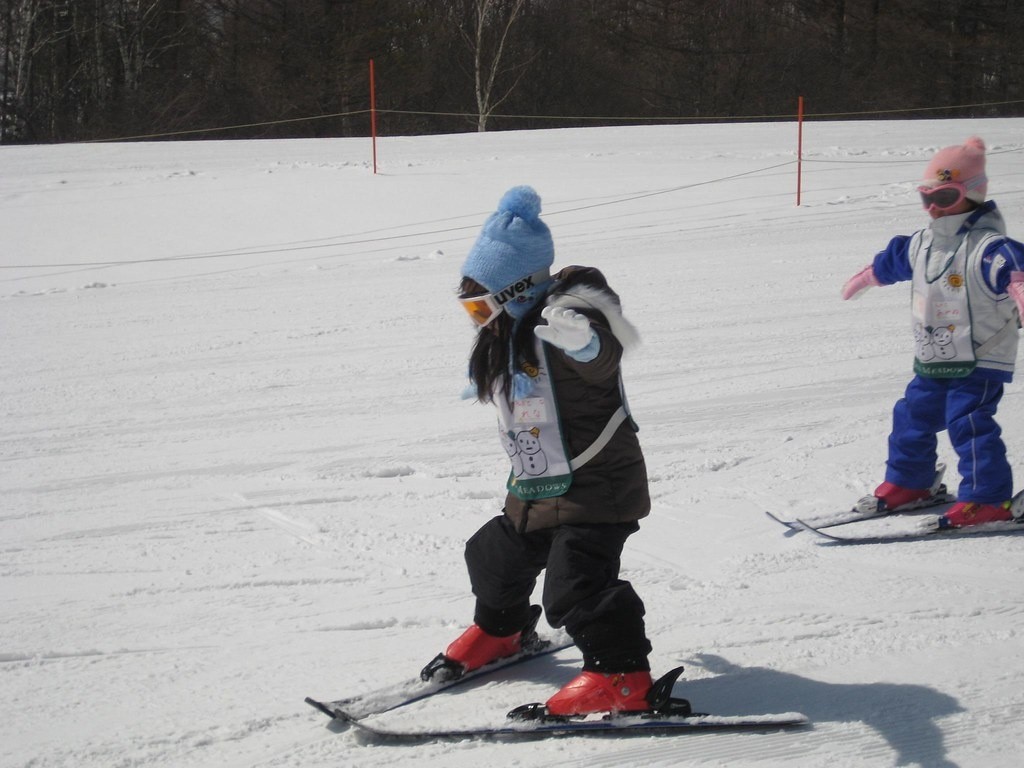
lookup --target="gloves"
[843,265,883,300]
[533,305,591,353]
[1006,272,1024,328]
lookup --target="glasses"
[458,293,504,328]
[916,183,966,211]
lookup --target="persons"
[444,186,655,718]
[838,136,1023,528]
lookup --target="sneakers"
[448,597,523,673]
[875,481,931,512]
[543,661,657,717]
[939,495,1012,528]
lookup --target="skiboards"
[304,623,811,734]
[765,492,1023,540]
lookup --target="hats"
[918,136,989,204]
[460,184,554,320]
